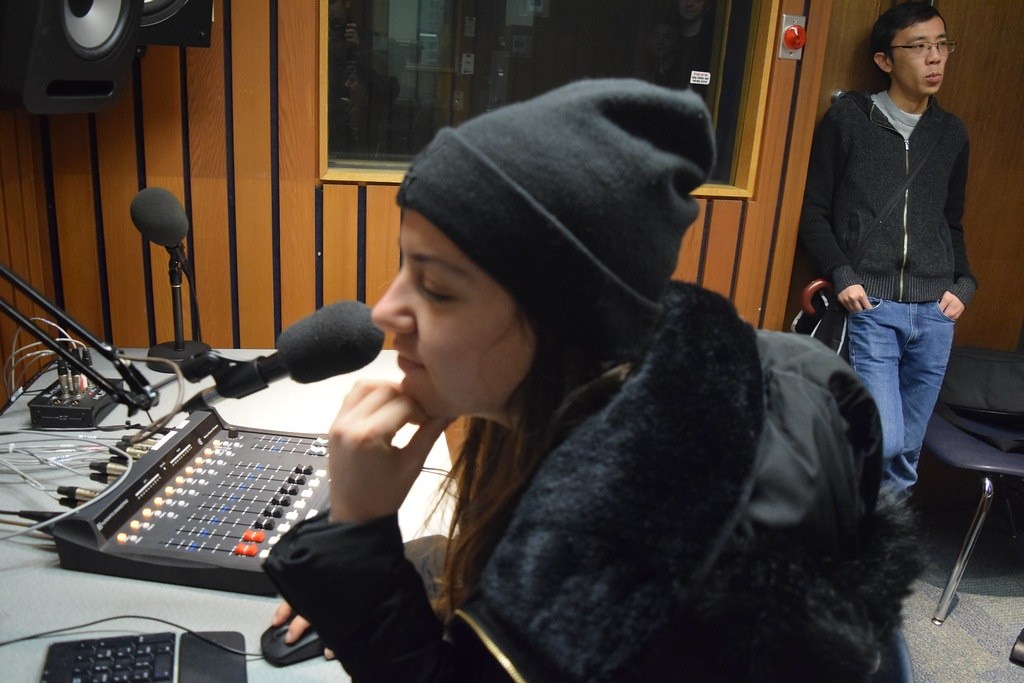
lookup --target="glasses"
[885,40,957,54]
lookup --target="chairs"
[924,410,1024,628]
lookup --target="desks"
[1,342,458,682]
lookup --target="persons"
[799,2,980,506]
[623,18,682,82]
[328,0,406,154]
[676,0,714,103]
[263,76,923,682]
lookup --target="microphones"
[131,188,193,282]
[180,300,385,414]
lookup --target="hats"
[397,77,715,350]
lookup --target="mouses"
[260,608,327,667]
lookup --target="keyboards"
[39,631,248,683]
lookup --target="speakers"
[142,0,214,48]
[0,0,145,114]
